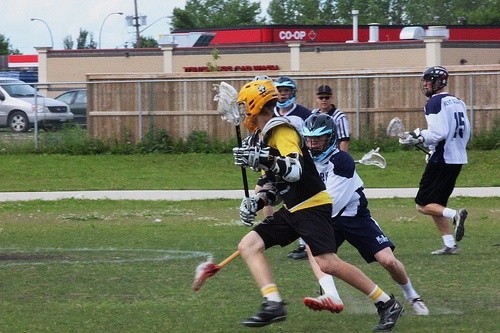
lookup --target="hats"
[315,84,333,98]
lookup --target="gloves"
[398,126,425,146]
[232,142,272,173]
[239,194,260,227]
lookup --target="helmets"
[300,112,338,154]
[419,65,449,98]
[235,74,280,133]
[274,75,298,109]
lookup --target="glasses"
[318,96,330,100]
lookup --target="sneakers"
[430,244,459,255]
[452,208,468,242]
[241,296,290,327]
[286,244,309,260]
[404,292,430,316]
[370,293,406,332]
[303,294,344,313]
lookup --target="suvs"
[0,68,39,88]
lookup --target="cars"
[0,77,75,134]
[41,89,89,134]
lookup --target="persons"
[398,66,470,256]
[233,74,431,332]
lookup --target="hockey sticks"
[190,250,240,291]
[384,117,431,156]
[213,81,251,219]
[352,147,390,170]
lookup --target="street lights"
[30,18,54,52]
[133,0,175,47]
[98,12,124,49]
